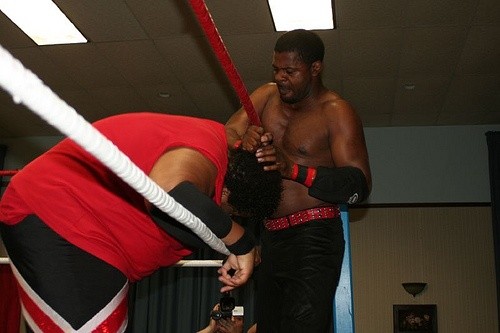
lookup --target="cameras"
[211,295,244,321]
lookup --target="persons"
[194,302,245,333]
[246,322,257,333]
[0,111,284,333]
[224,29,374,333]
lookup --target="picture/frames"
[393,304,438,333]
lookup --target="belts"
[262,205,340,232]
